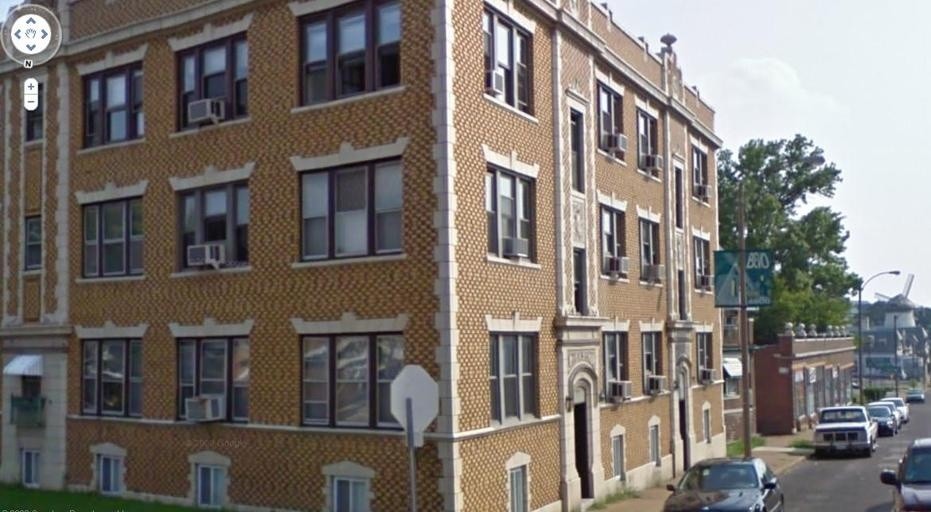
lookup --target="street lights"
[857,272,897,401]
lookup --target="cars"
[813,396,908,455]
[906,390,925,402]
[880,438,931,511]
[664,459,784,512]
